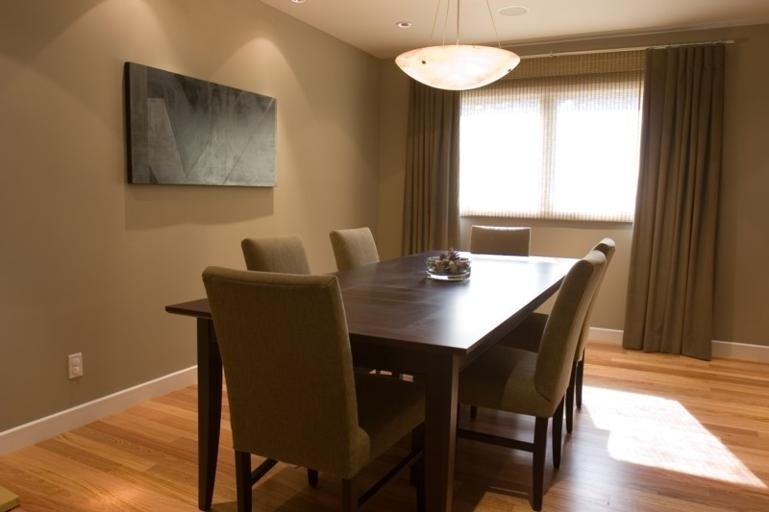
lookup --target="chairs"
[329,227,390,267]
[470,226,533,254]
[202,258,426,512]
[469,237,616,433]
[237,238,328,274]
[460,249,606,510]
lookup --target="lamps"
[394,0,523,95]
